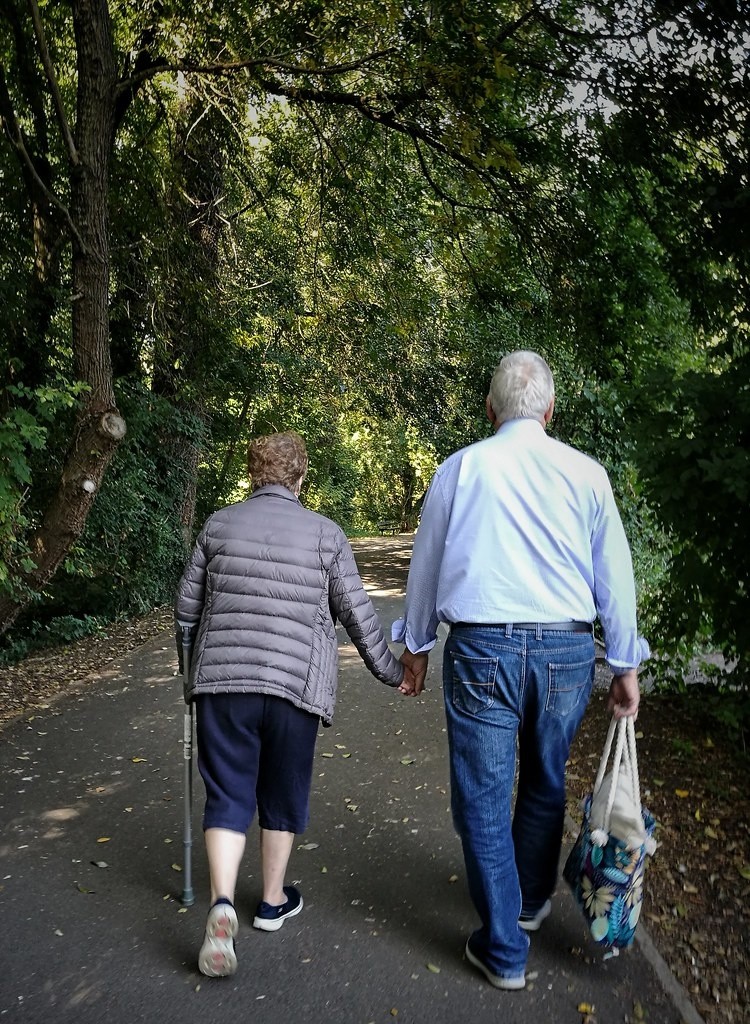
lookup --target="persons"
[390,350,652,989]
[175,429,427,978]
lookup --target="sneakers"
[465,934,526,990]
[518,899,552,930]
[252,886,304,932]
[198,898,238,977]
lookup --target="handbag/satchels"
[561,707,657,962]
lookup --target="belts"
[451,621,592,632]
